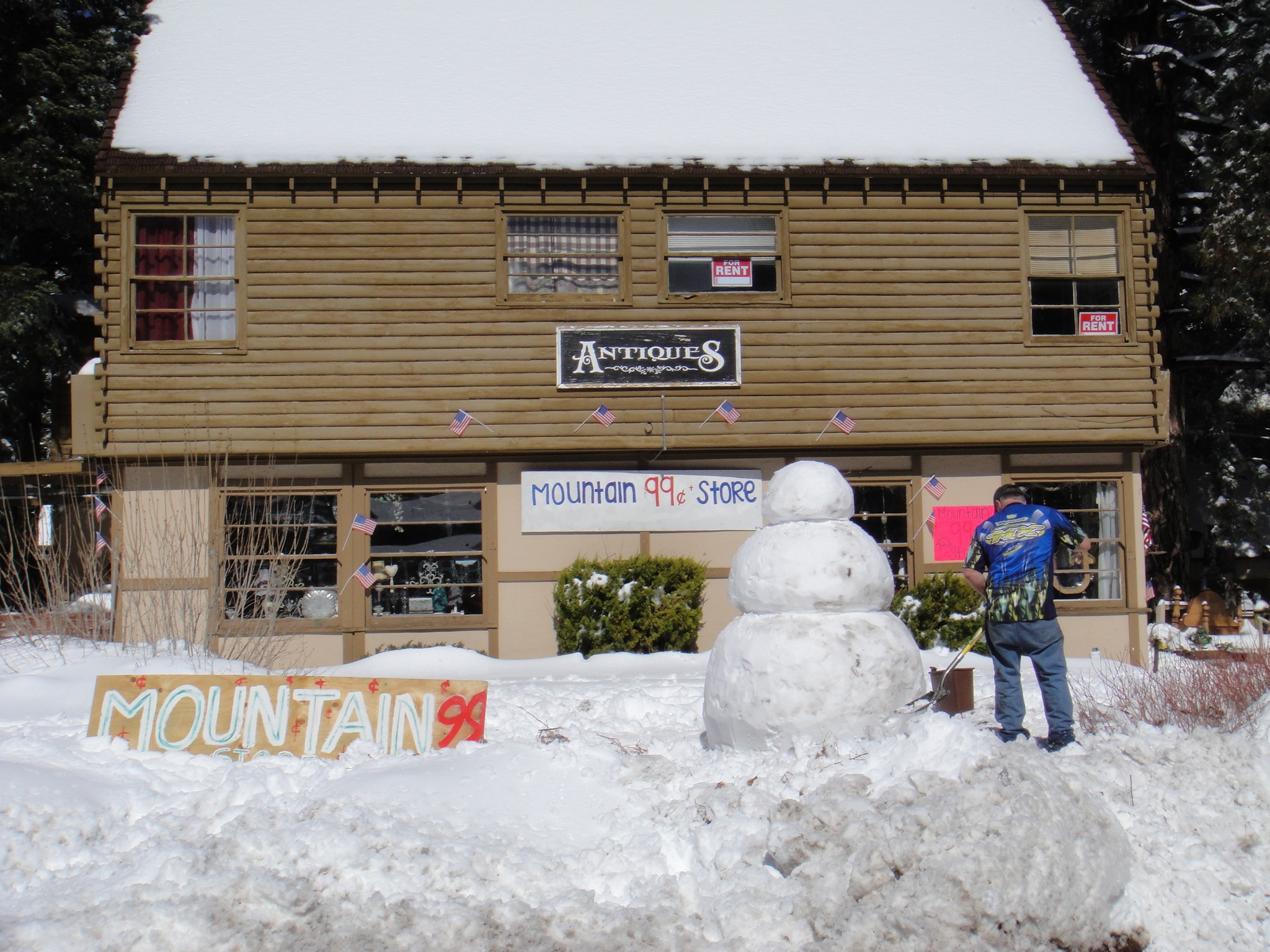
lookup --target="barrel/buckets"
[928,666,974,717]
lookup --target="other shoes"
[1047,730,1075,752]
[999,727,1029,741]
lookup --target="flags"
[93,467,109,489]
[927,511,937,531]
[92,498,106,521]
[92,535,107,558]
[354,563,379,589]
[593,403,617,428]
[924,475,948,500]
[352,513,378,537]
[716,400,741,426]
[1143,500,1154,555]
[447,411,473,437]
[1146,579,1156,602]
[831,410,857,435]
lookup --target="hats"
[993,483,1023,514]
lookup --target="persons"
[963,483,1091,755]
